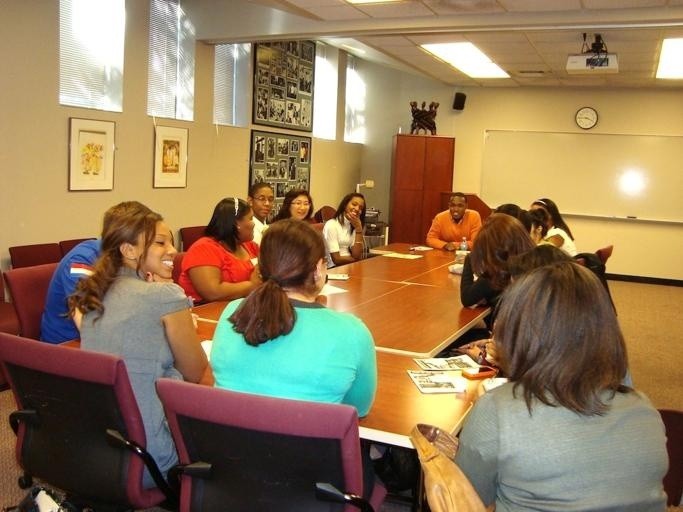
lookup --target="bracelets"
[355,231,363,234]
[355,242,363,244]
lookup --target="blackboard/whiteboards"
[479,129,683,224]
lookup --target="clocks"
[575,106,598,129]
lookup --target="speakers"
[453,93,466,110]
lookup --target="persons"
[209,219,378,502]
[454,261,669,511]
[271,187,313,219]
[492,203,531,237]
[321,193,365,267]
[531,198,578,257]
[41,201,149,345]
[506,245,572,283]
[460,212,536,309]
[58,207,208,491]
[412,353,478,369]
[248,182,275,275]
[528,208,557,246]
[180,198,261,307]
[426,191,482,251]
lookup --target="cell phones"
[463,365,500,379]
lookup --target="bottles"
[460,237,468,251]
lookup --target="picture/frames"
[153,125,190,189]
[249,128,313,225]
[66,117,116,191]
[254,40,316,134]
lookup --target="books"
[320,285,348,294]
[407,370,464,394]
[382,252,424,260]
[412,246,433,251]
[328,273,349,281]
[456,250,467,256]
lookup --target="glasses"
[285,201,312,207]
[252,196,274,202]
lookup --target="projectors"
[566,53,618,74]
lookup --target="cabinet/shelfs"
[388,132,456,245]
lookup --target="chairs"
[1,330,176,510]
[7,243,62,268]
[60,238,97,261]
[179,225,209,251]
[3,262,59,341]
[153,375,388,511]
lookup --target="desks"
[56,240,507,511]
